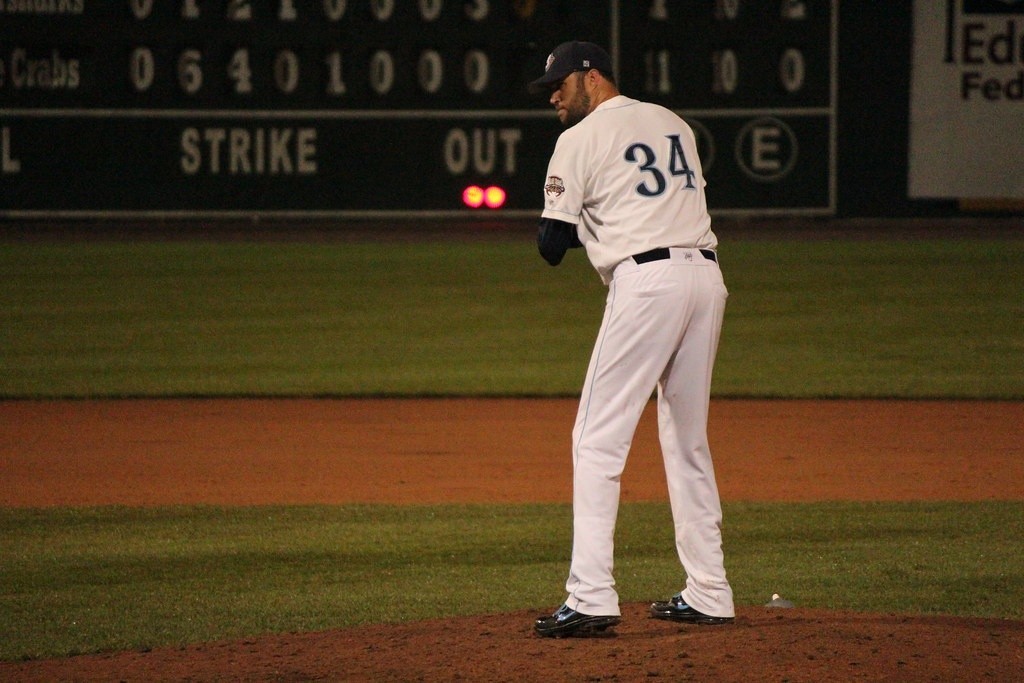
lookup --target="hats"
[531,40,616,104]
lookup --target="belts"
[631,248,718,265]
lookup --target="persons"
[527,40,737,634]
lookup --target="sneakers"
[648,593,735,624]
[532,601,622,636]
